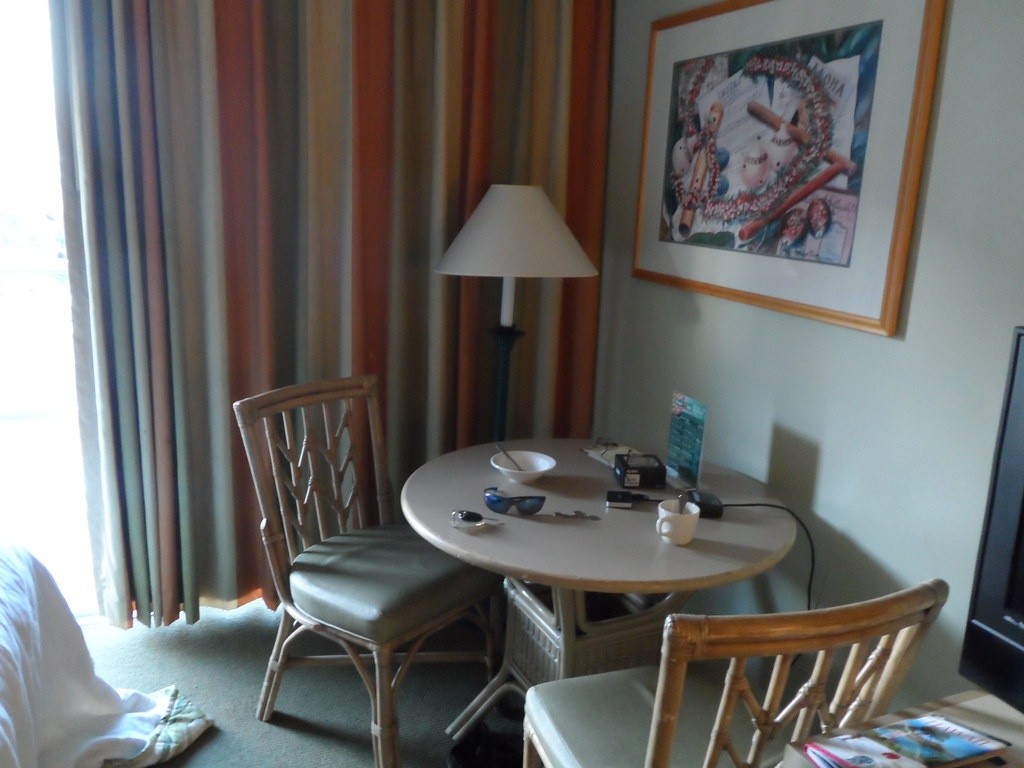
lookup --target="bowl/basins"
[490,451,557,483]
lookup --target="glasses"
[483,487,546,516]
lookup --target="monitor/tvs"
[960,328,1024,714]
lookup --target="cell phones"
[605,490,633,508]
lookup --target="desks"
[400,438,797,742]
[785,686,1024,768]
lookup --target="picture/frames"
[631,0,943,336]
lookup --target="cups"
[655,500,700,546]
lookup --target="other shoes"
[447,721,523,767]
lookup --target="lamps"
[435,181,600,443]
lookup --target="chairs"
[523,578,949,768]
[232,374,503,768]
